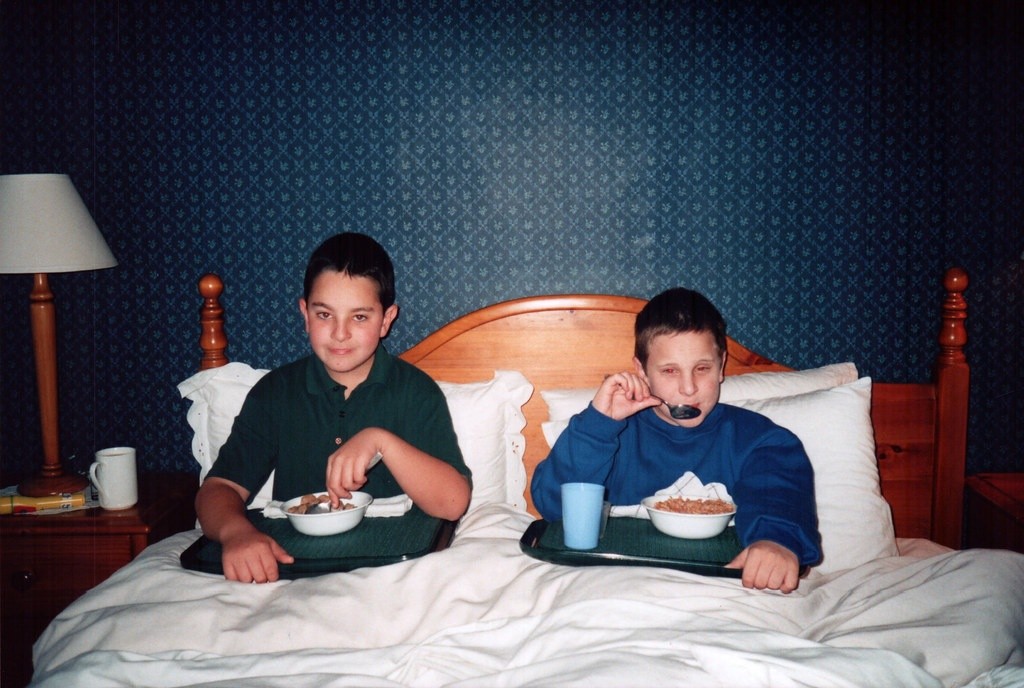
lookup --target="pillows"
[541,362,857,422]
[542,376,900,576]
[177,361,534,515]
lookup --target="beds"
[30,230,1024,688]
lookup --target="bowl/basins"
[280,491,373,537]
[641,494,737,539]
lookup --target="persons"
[195,232,473,585]
[529,286,819,594]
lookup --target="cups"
[89,447,139,511]
[561,482,605,551]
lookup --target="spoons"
[305,452,382,514]
[604,373,701,419]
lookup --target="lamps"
[0,172,117,498]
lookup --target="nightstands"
[964,472,1024,555]
[0,472,199,688]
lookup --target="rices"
[653,497,734,515]
